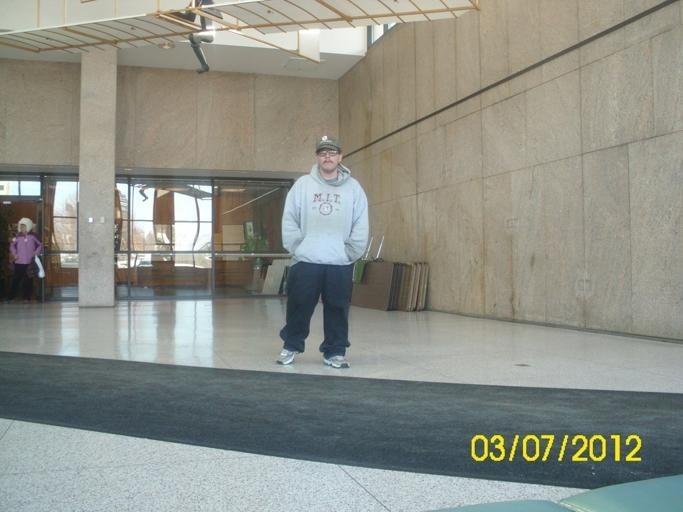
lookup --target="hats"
[316,135,341,152]
[17,217,33,233]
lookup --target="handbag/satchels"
[32,254,45,279]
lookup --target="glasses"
[317,150,338,156]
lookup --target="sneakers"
[323,355,350,369]
[276,349,300,366]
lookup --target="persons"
[9,217,41,302]
[275,136,368,367]
